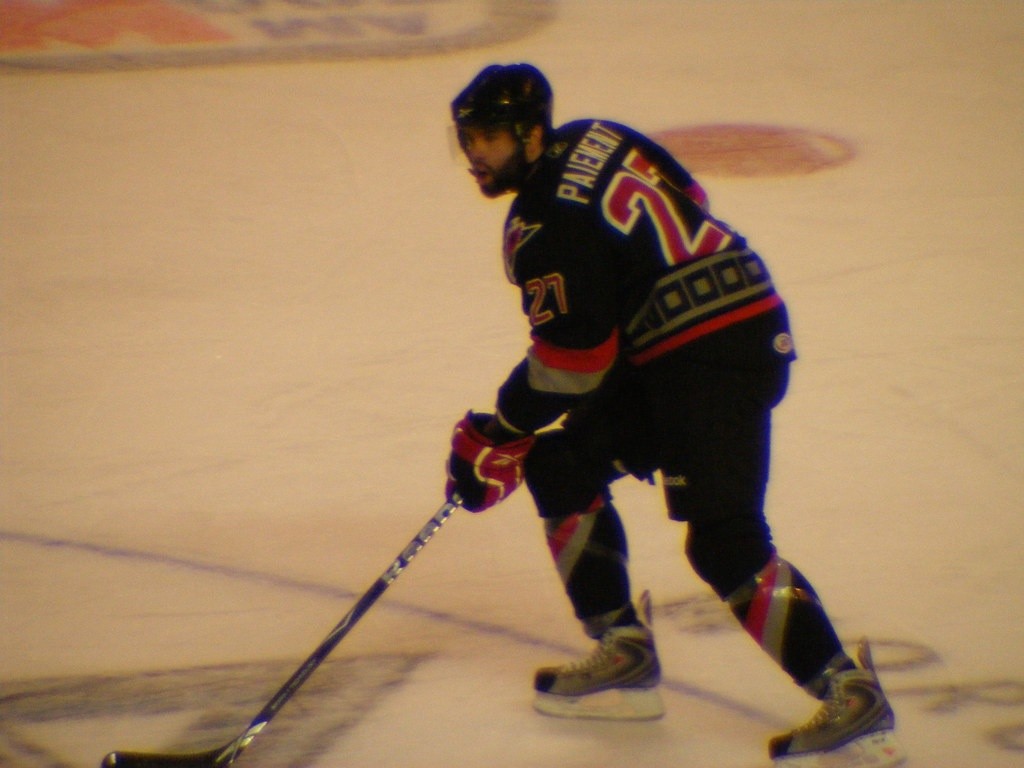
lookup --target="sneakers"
[526,590,666,721]
[768,636,904,768]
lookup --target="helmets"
[450,63,552,144]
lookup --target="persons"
[445,64,895,759]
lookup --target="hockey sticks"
[99,489,465,768]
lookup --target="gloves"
[445,410,536,514]
